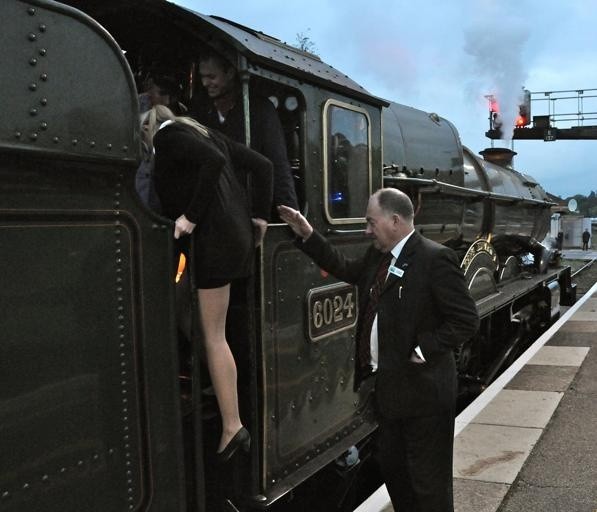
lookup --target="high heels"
[211,427,250,468]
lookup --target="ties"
[357,250,394,369]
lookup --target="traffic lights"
[533,116,549,128]
[492,97,502,129]
[516,90,530,125]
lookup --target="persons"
[138,105,273,464]
[190,49,299,216]
[583,228,590,251]
[277,188,482,512]
[135,64,188,218]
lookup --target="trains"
[0,0,582,511]
